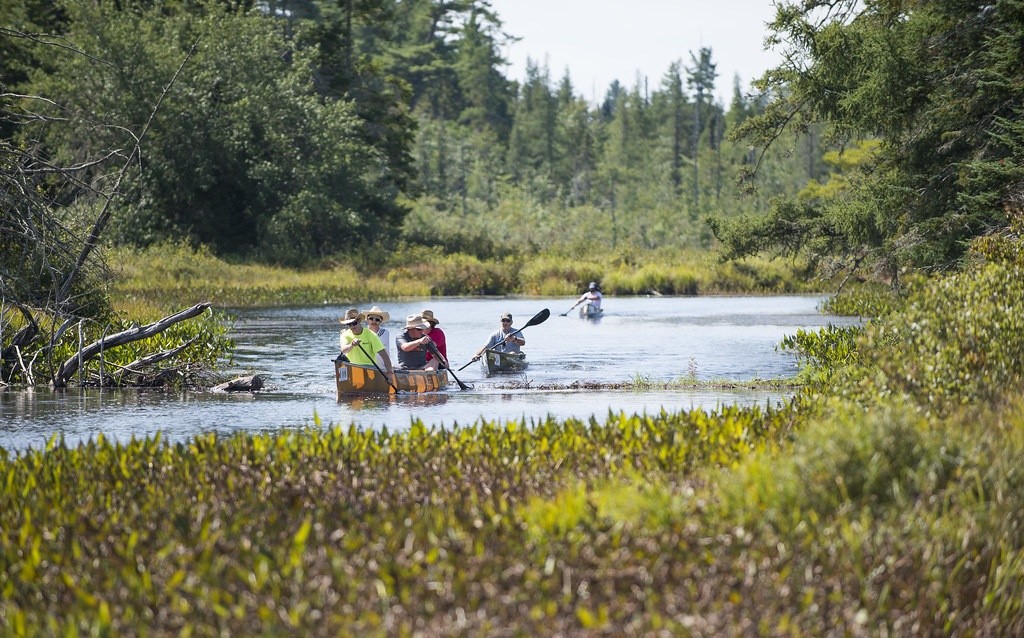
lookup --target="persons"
[577,282,602,312]
[472,312,526,362]
[338,305,449,386]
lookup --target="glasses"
[415,328,422,330]
[502,319,510,322]
[347,321,357,327]
[368,317,381,322]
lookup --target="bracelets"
[387,371,394,374]
[512,337,517,342]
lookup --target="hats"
[339,308,362,325]
[361,306,390,323]
[401,315,430,330]
[420,310,439,324]
[589,282,597,289]
[500,313,512,321]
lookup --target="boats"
[479,349,530,378]
[579,304,603,319]
[330,359,448,396]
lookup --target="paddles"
[356,342,402,396]
[455,308,551,372]
[557,296,588,318]
[426,340,473,392]
